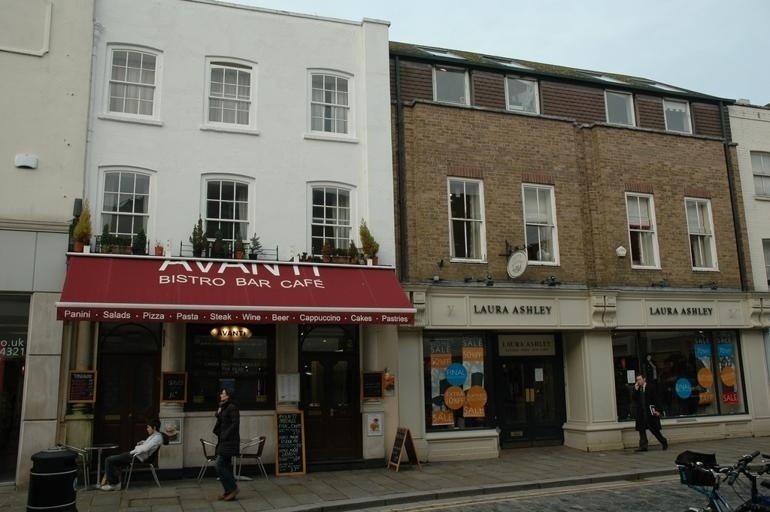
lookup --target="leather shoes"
[634,439,667,451]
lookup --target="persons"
[632,374,669,451]
[95,418,164,491]
[212,386,240,500]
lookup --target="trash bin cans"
[26,447,82,512]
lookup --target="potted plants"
[321,216,379,266]
[188,212,264,259]
[72,198,164,256]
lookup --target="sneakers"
[101,483,121,491]
[218,488,240,501]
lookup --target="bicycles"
[675,445,770,512]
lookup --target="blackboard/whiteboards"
[388,428,408,466]
[160,371,187,404]
[68,370,97,404]
[361,369,386,400]
[274,409,307,475]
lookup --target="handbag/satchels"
[675,451,717,487]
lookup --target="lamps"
[499,239,528,280]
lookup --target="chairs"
[123,444,161,492]
[55,442,89,491]
[236,435,269,482]
[197,438,217,485]
